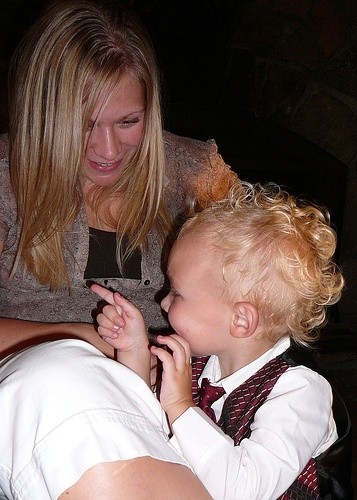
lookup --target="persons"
[90,181,343,500]
[0,0,248,500]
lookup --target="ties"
[198,377,226,423]
